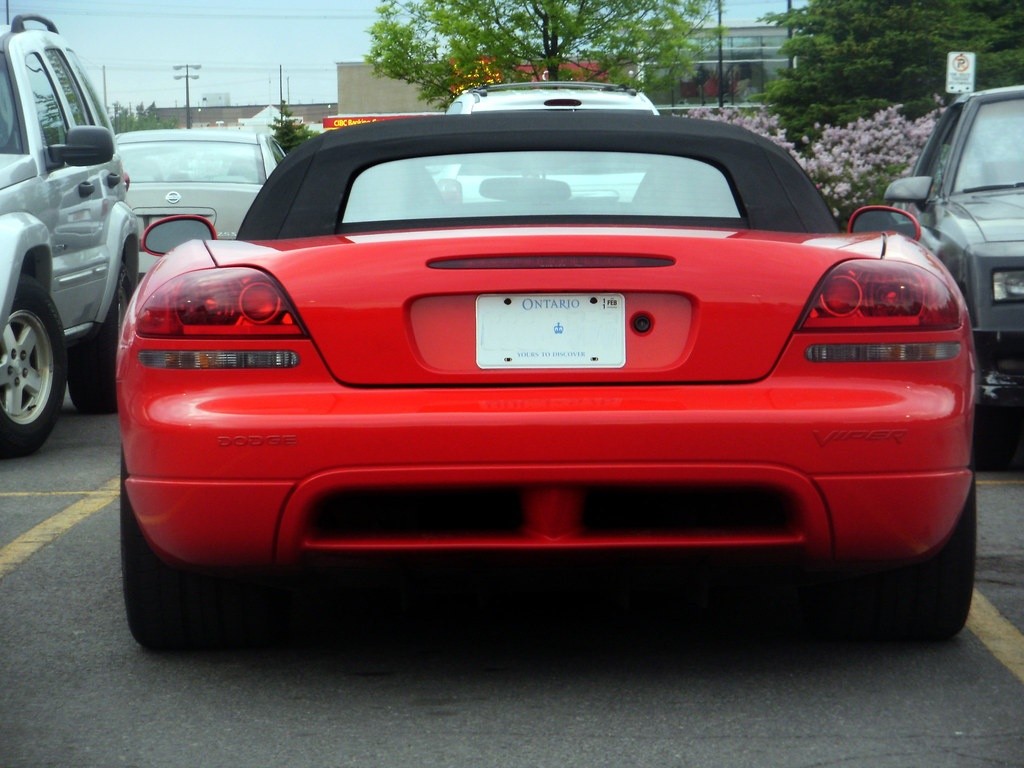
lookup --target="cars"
[107,122,286,283]
[889,84,1024,476]
[436,80,660,206]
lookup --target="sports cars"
[114,113,980,661]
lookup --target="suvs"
[1,10,142,460]
